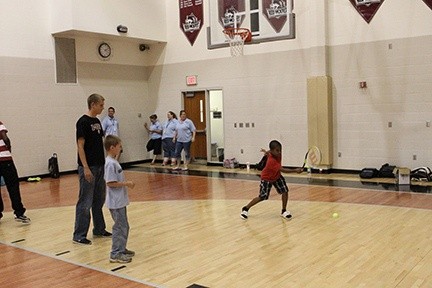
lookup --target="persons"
[172,110,197,171]
[1,114,31,223]
[161,112,179,166]
[104,135,136,262]
[144,115,163,165]
[73,94,113,245]
[242,140,292,219]
[101,107,123,161]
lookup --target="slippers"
[280,209,292,220]
[240,207,249,219]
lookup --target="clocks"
[96,41,114,61]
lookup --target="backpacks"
[256,150,268,171]
[48,153,60,178]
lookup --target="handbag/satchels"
[379,163,395,189]
[224,158,238,169]
[395,167,410,184]
[360,167,378,185]
[411,167,432,192]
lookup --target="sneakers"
[121,250,134,257]
[110,254,132,263]
[93,231,113,236]
[14,215,30,224]
[72,237,91,245]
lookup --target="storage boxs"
[392,167,411,184]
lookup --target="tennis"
[332,213,339,219]
